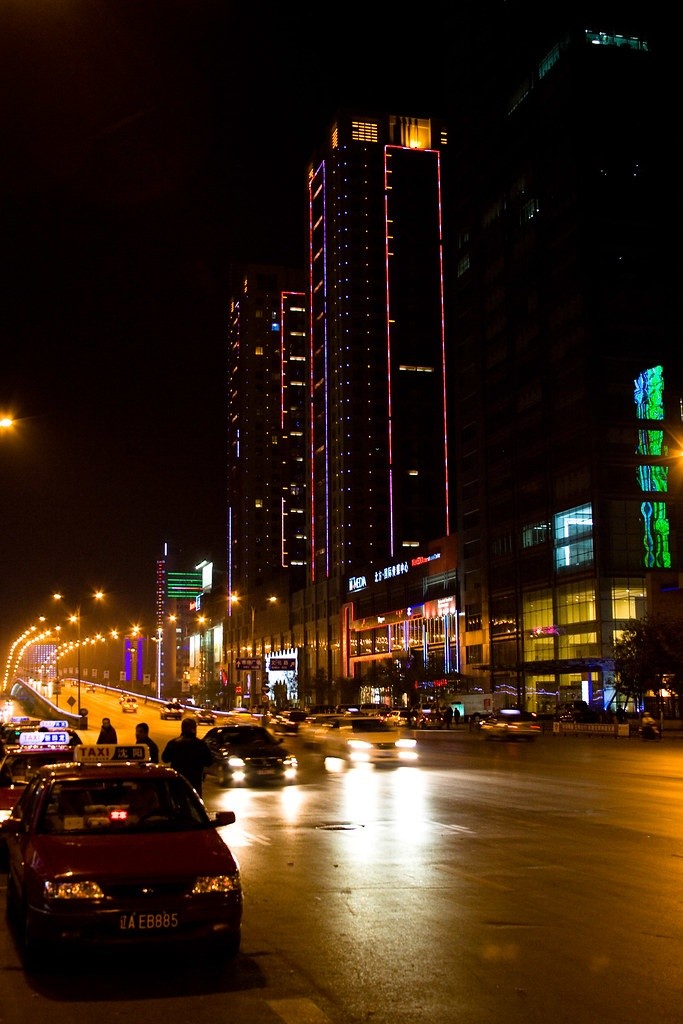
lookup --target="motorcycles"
[639,726,662,741]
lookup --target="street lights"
[53,592,104,713]
[2,617,61,707]
[131,624,156,696]
[54,628,119,686]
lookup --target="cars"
[480,710,543,741]
[0,744,243,977]
[201,724,298,786]
[336,702,444,730]
[0,718,84,822]
[196,711,217,725]
[159,703,183,720]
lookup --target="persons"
[436,709,443,721]
[444,707,453,728]
[96,717,117,747]
[605,708,614,725]
[161,718,214,808]
[642,711,656,729]
[133,723,158,765]
[616,704,624,725]
[454,708,460,724]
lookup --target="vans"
[122,697,139,713]
